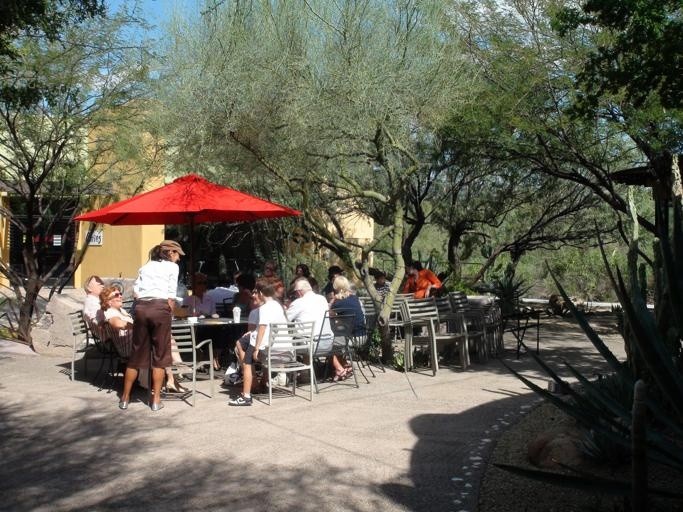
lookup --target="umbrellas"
[73,172,306,290]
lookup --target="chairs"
[70,297,354,406]
[357,290,542,377]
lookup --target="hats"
[328,267,343,273]
[161,240,186,256]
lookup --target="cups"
[232,304,242,323]
[187,316,198,323]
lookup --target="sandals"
[332,365,353,381]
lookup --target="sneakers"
[229,373,289,405]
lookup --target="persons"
[289,264,319,298]
[322,266,346,303]
[404,261,441,300]
[119,238,187,412]
[228,274,368,406]
[177,271,242,372]
[83,275,193,393]
[374,271,391,296]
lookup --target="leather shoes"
[119,367,193,411]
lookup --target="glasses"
[108,293,123,300]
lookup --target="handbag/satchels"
[430,285,454,311]
